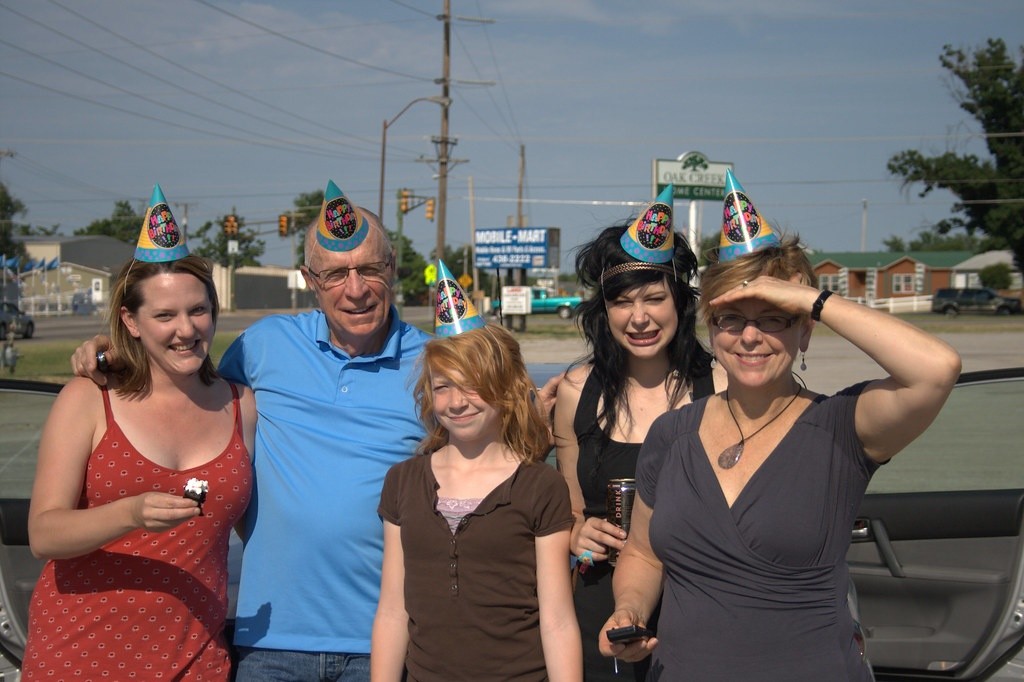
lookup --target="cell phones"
[606,624,653,644]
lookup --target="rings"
[744,280,747,286]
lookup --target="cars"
[931,287,1020,317]
[0,301,35,340]
[0,366,1024,679]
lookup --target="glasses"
[710,312,804,331]
[306,250,393,287]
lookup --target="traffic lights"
[224,215,239,238]
[279,215,288,236]
[425,197,434,222]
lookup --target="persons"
[20,256,258,682]
[369,321,583,682]
[70,205,565,682]
[598,230,961,682]
[552,224,728,682]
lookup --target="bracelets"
[811,290,833,321]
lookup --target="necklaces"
[718,383,802,469]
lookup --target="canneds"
[605,478,636,567]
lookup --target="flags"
[0,256,58,271]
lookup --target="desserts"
[184,477,209,517]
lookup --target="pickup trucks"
[490,287,584,319]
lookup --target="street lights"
[378,97,451,230]
